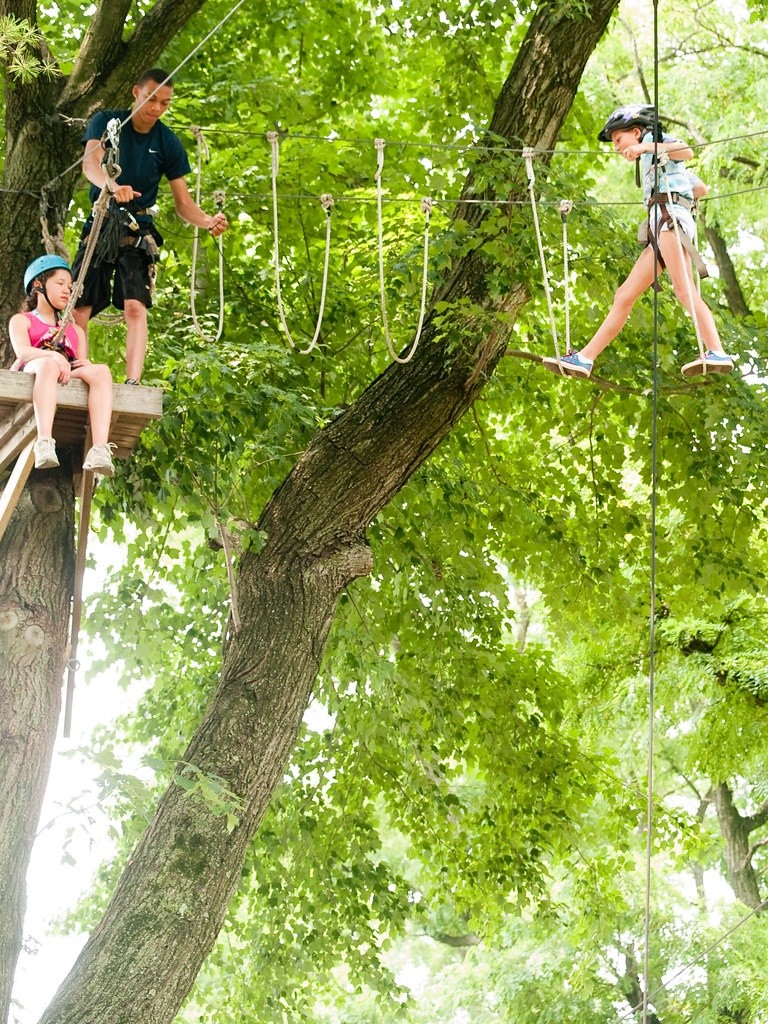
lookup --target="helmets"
[24,255,72,296]
[597,103,654,142]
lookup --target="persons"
[542,103,734,378]
[65,69,230,383]
[8,254,115,476]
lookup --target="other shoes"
[125,378,139,386]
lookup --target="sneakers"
[543,354,593,378]
[33,437,59,469]
[681,351,735,377]
[82,442,119,476]
